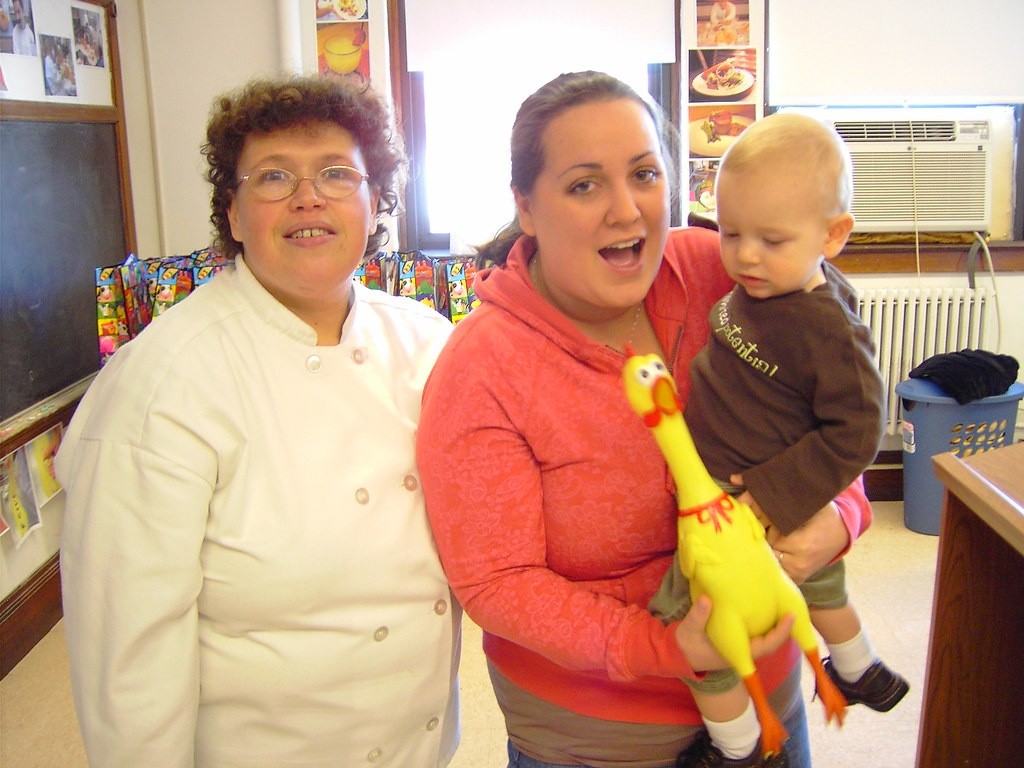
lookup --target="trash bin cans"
[894,377,1024,536]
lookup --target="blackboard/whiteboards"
[0,97,149,445]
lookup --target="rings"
[779,550,784,562]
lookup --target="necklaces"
[530,254,641,347]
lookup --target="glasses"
[233,165,370,201]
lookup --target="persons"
[43,37,76,96]
[77,35,106,68]
[55,72,461,768]
[709,0,737,32]
[44,426,61,479]
[414,70,874,768]
[645,112,909,768]
[11,0,37,57]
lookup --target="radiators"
[854,284,997,436]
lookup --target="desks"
[912,445,1023,767]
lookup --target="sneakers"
[811,655,908,712]
[674,728,791,768]
